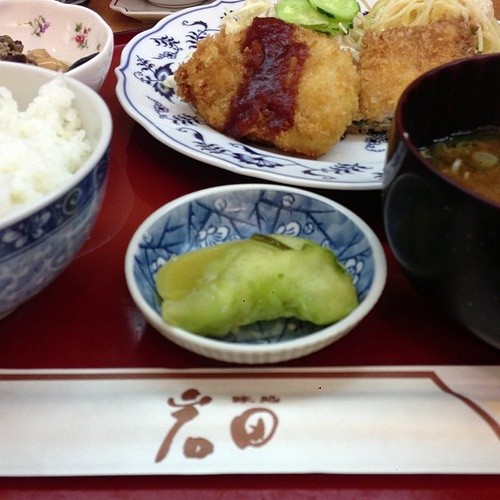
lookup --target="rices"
[0,73,90,224]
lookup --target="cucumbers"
[275,0,360,35]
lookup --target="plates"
[114,0,500,190]
[109,0,215,20]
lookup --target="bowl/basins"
[124,184,386,362]
[382,52,500,350]
[0,61,112,317]
[0,0,114,93]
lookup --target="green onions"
[417,138,499,187]
